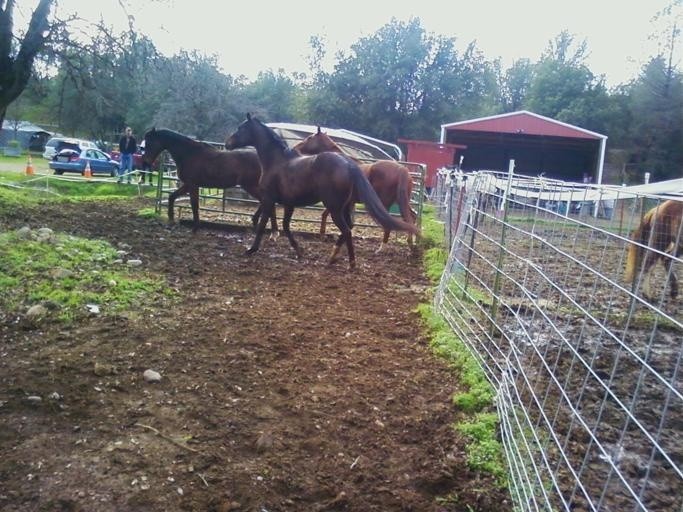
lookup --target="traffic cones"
[26,155,33,176]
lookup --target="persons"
[117,127,152,186]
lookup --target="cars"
[42,137,176,177]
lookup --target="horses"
[223,111,423,273]
[141,124,281,247]
[291,123,420,256]
[622,197,683,306]
